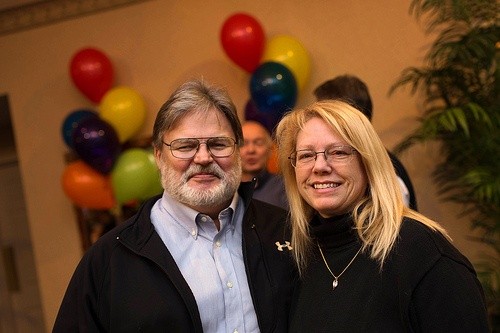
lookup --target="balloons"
[110,148,163,203]
[69,46,115,104]
[61,109,99,150]
[74,116,120,176]
[264,34,310,92]
[266,144,283,174]
[61,159,115,209]
[220,13,266,75]
[102,85,144,143]
[250,60,299,115]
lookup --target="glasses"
[286,145,359,169]
[161,135,239,158]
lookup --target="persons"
[312,73,418,215]
[52,80,297,333]
[276,100,490,333]
[239,123,292,213]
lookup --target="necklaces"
[315,238,364,290]
